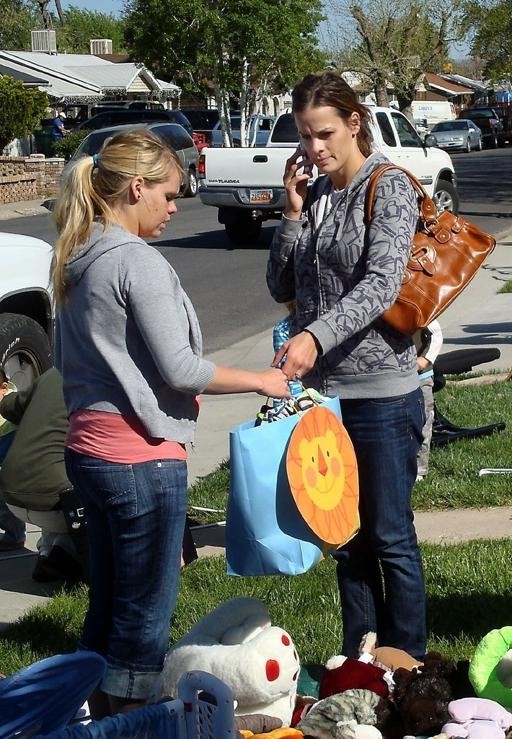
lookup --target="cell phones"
[298,138,313,173]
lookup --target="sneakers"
[33,549,67,583]
[0,533,26,551]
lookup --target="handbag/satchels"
[226,379,361,576]
[363,162,496,338]
[59,489,89,555]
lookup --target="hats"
[59,112,67,118]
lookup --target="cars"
[429,106,512,153]
[0,231,56,392]
[60,110,278,198]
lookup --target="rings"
[294,371,302,379]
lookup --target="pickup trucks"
[198,106,460,242]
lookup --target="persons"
[270,73,423,658]
[45,130,290,715]
[0,367,29,550]
[413,319,442,483]
[270,295,308,402]
[50,111,68,142]
[0,368,89,584]
